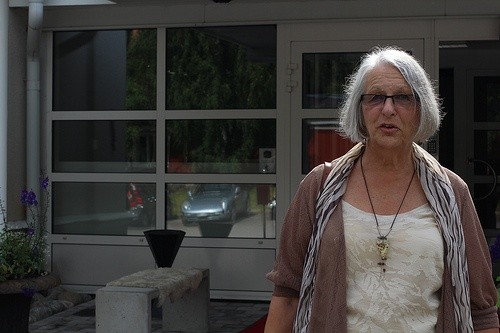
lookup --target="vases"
[0,272,49,333]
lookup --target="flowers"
[0,176,52,299]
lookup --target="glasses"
[359,91,420,111]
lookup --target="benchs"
[95,268,210,333]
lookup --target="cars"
[179,183,251,227]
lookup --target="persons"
[263,48,500,333]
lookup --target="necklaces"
[360,153,416,273]
